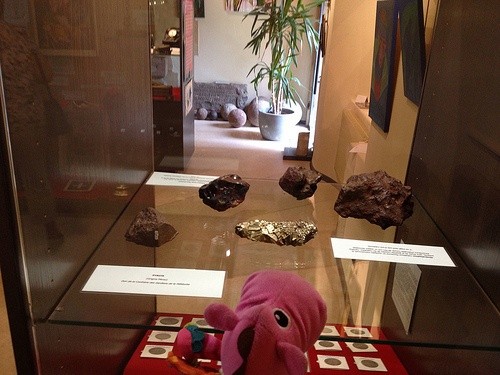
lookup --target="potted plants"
[242,0,328,140]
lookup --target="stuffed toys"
[168,269,327,375]
